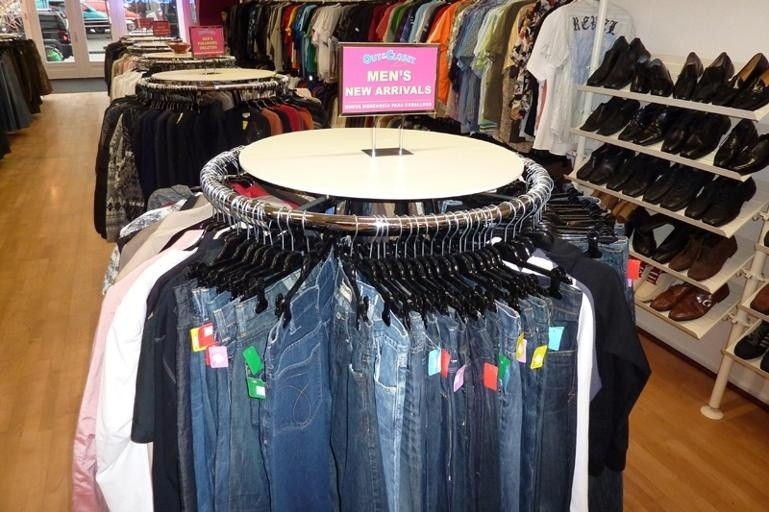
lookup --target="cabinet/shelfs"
[572,41,765,425]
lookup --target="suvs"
[38,11,72,60]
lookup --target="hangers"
[114,73,313,118]
[154,127,620,322]
[115,31,236,74]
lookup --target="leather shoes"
[607,153,652,192]
[649,283,690,311]
[624,208,657,257]
[605,37,651,91]
[580,96,622,134]
[687,234,738,282]
[589,147,634,186]
[668,231,711,271]
[682,113,731,161]
[587,36,628,86]
[729,133,769,176]
[714,119,756,167]
[735,67,769,110]
[684,174,728,220]
[631,53,651,94]
[750,280,769,313]
[662,111,701,154]
[660,169,714,210]
[672,52,703,101]
[596,99,640,137]
[647,213,693,263]
[576,142,611,184]
[623,157,670,197]
[651,57,673,97]
[692,52,734,105]
[668,280,730,321]
[711,53,766,105]
[702,177,756,226]
[619,103,666,141]
[642,163,685,203]
[632,107,678,146]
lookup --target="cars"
[81,0,110,32]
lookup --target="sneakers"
[632,262,653,292]
[632,267,677,303]
[759,349,769,373]
[733,318,769,359]
